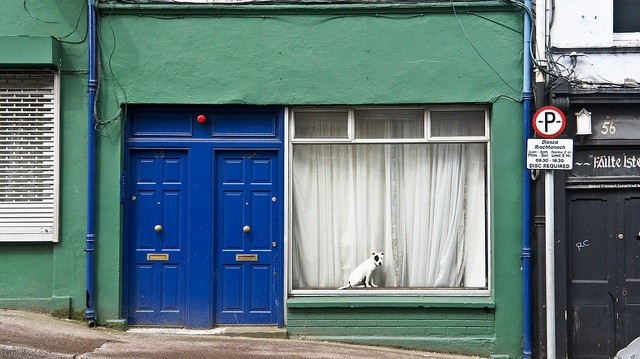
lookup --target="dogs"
[337,252,385,290]
